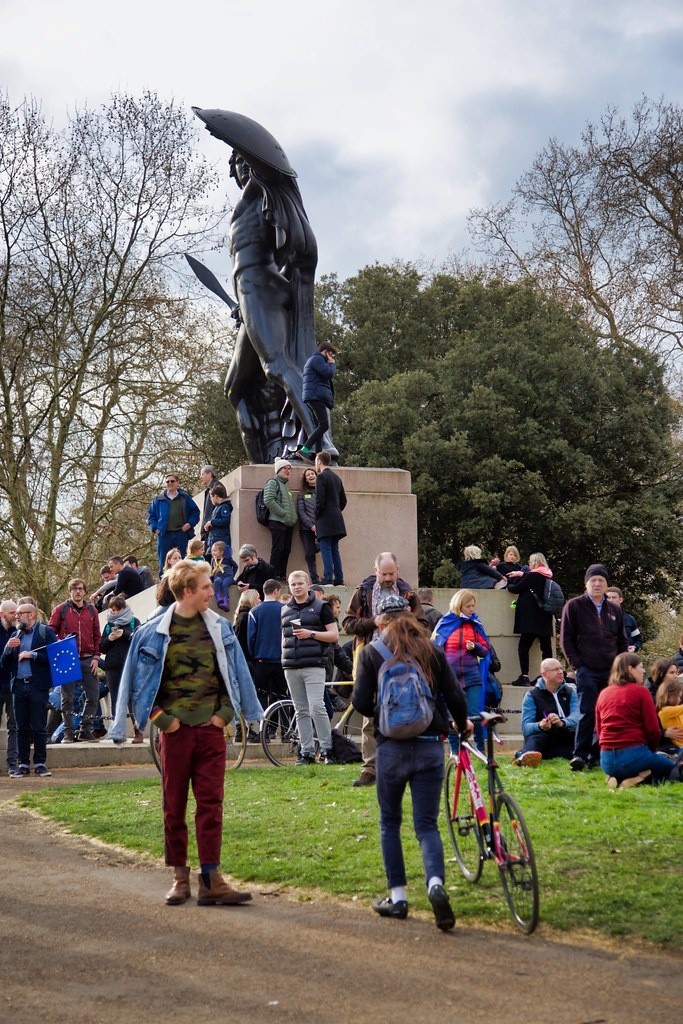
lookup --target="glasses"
[72,587,83,591]
[546,667,563,672]
[378,571,394,576]
[243,557,253,563]
[15,611,31,616]
[166,480,175,483]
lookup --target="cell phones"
[238,584,245,587]
[112,626,118,632]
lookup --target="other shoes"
[428,884,456,931]
[312,578,346,588]
[512,674,530,687]
[512,751,652,790]
[296,449,315,466]
[217,595,224,609]
[219,597,230,612]
[351,772,375,787]
[318,749,334,766]
[8,724,144,779]
[373,898,408,919]
[295,752,314,766]
[234,724,291,744]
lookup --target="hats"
[584,563,608,585]
[377,596,409,612]
[275,457,292,474]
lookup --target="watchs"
[311,630,316,639]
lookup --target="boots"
[196,870,252,905]
[165,866,191,905]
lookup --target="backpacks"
[370,638,434,738]
[530,578,566,614]
[255,478,280,527]
[331,729,362,764]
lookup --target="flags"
[46,636,84,687]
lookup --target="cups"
[290,618,301,631]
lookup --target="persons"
[191,104,339,463]
[559,563,628,771]
[106,557,264,907]
[594,651,673,793]
[262,455,298,584]
[146,473,201,580]
[280,568,340,766]
[506,551,553,687]
[350,594,474,934]
[1,340,683,792]
[294,340,338,465]
[512,657,580,770]
[313,451,348,587]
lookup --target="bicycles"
[445,708,539,935]
[149,705,248,778]
[259,659,365,767]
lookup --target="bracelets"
[31,651,36,657]
[91,655,101,660]
